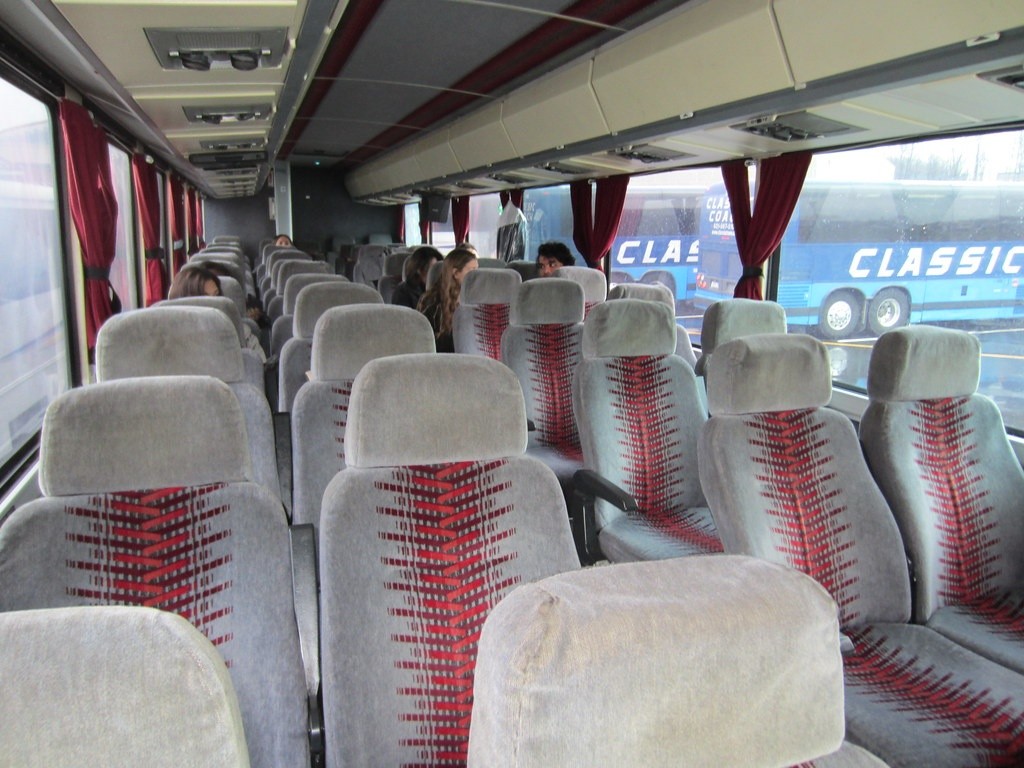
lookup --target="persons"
[416,243,478,354]
[272,234,291,246]
[536,242,575,278]
[391,247,444,309]
[167,260,268,369]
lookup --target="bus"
[693,181,1024,340]
[498,185,708,301]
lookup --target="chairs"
[0,237,1024,767]
